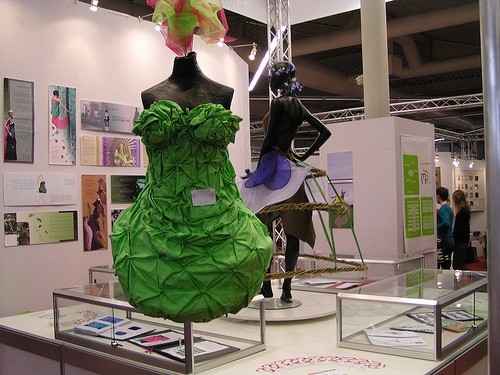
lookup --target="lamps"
[249,48,257,60]
[90,0,99,11]
[155,21,162,31]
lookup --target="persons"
[239,62,331,303]
[108,51,273,324]
[4,109,17,160]
[436,187,454,270]
[52,89,70,120]
[452,190,471,289]
